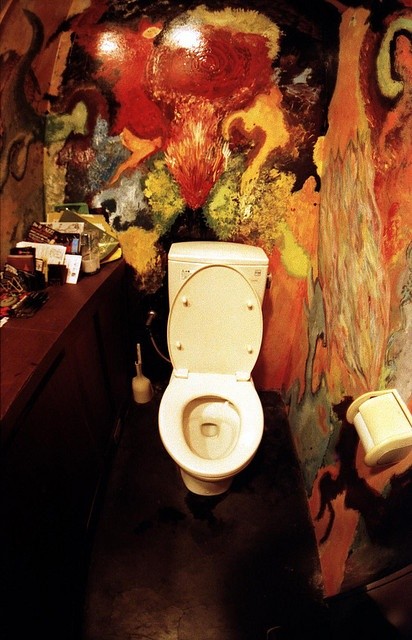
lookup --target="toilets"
[158,241,271,495]
